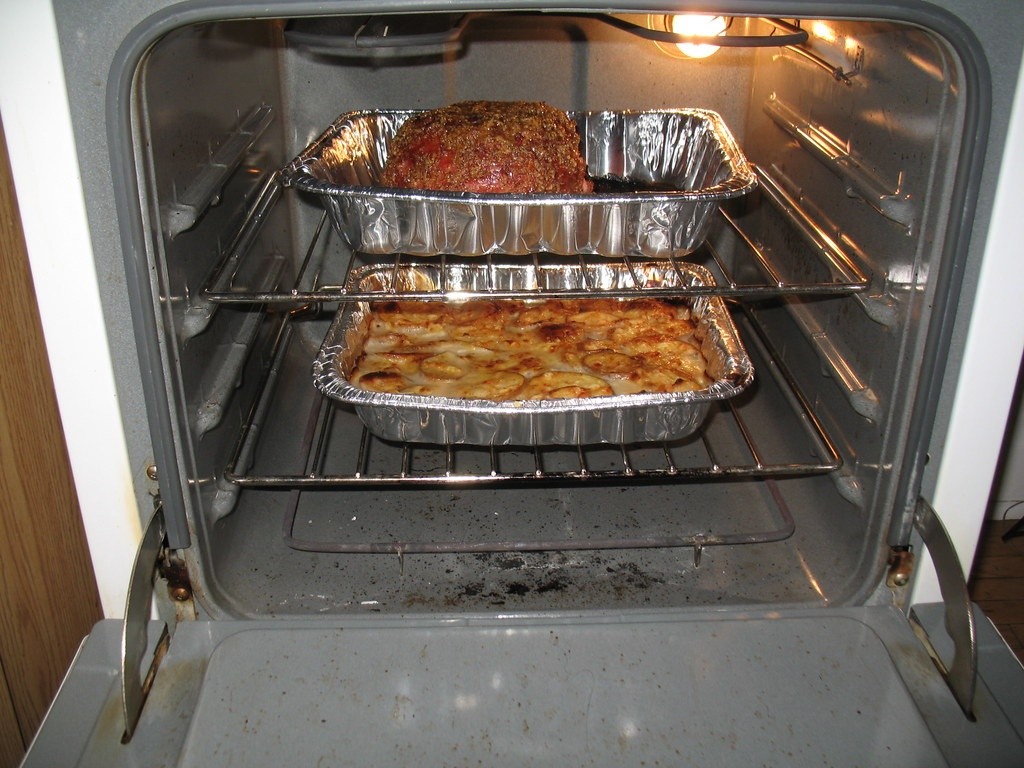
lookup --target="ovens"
[0,0,1024,768]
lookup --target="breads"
[383,102,583,195]
